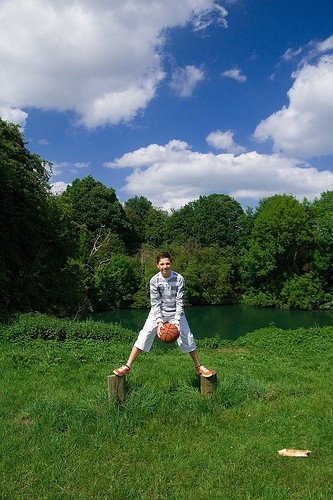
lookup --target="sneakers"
[195,365,213,378]
[112,365,130,377]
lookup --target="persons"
[112,251,213,378]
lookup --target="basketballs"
[160,323,179,344]
